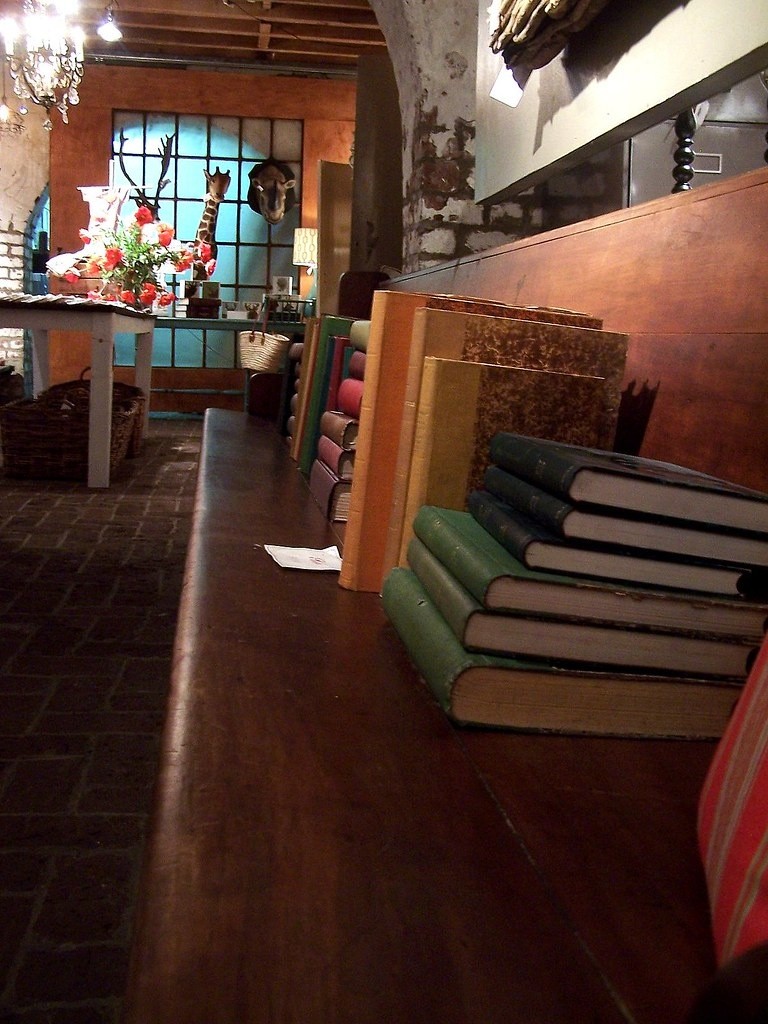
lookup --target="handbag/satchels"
[239,297,290,374]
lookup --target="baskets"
[37,367,145,458]
[0,394,134,480]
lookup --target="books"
[291,290,768,742]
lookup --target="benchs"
[117,163,768,1024]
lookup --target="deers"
[119,127,176,221]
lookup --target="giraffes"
[192,167,231,280]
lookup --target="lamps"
[0,0,88,132]
[292,228,318,269]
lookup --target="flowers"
[64,205,217,313]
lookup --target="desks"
[0,294,160,491]
